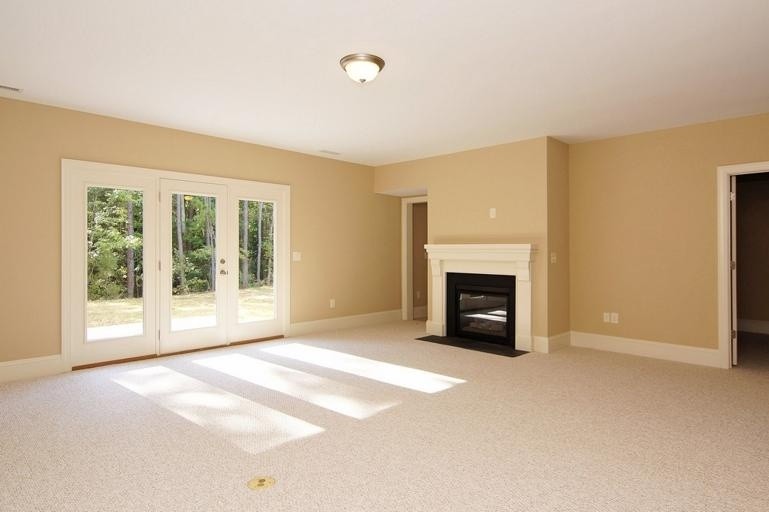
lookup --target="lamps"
[338,53,386,87]
